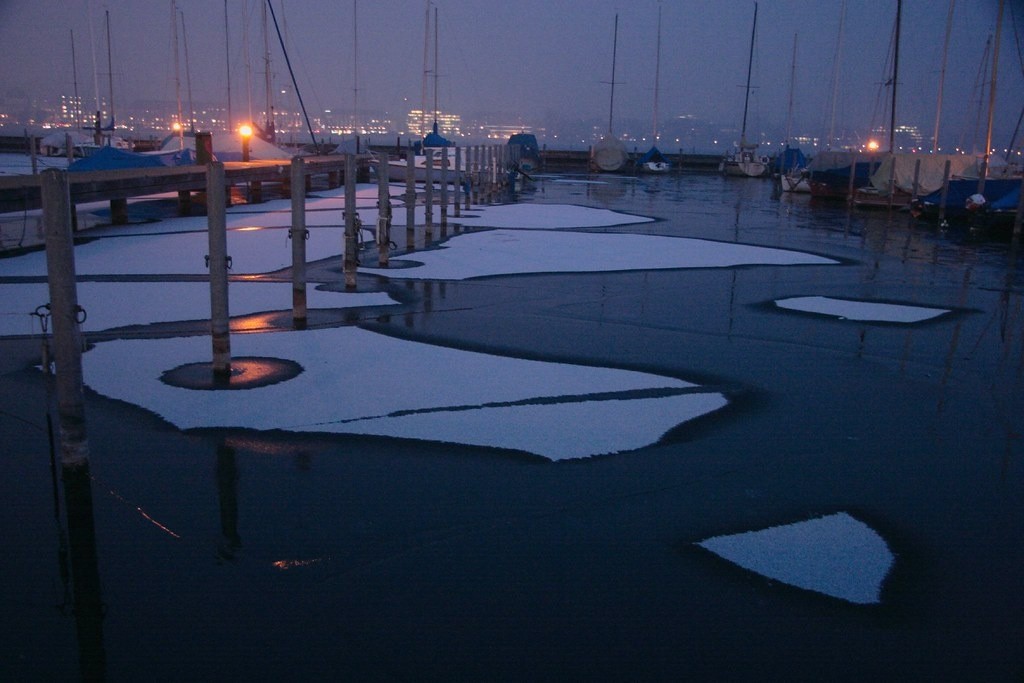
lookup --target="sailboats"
[326,1,543,158]
[718,1,1023,206]
[636,5,672,172]
[590,12,629,173]
[1,0,328,171]
